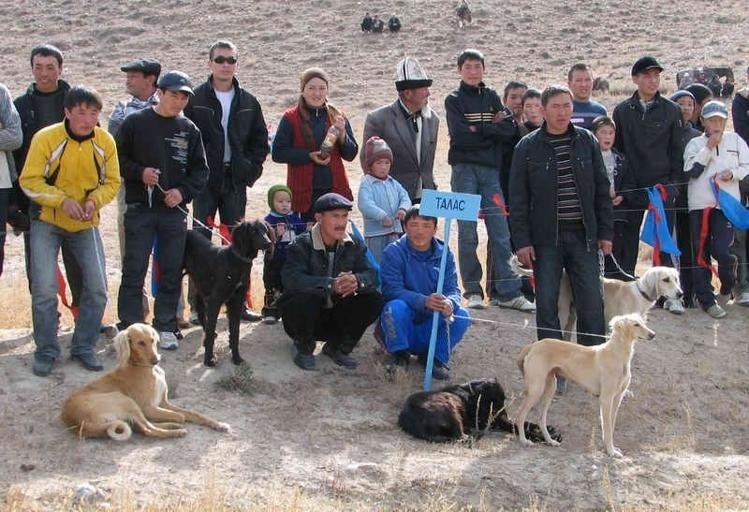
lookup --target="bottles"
[318,124,340,161]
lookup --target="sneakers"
[159,332,178,350]
[418,355,450,379]
[469,295,483,308]
[707,304,726,318]
[34,357,53,376]
[72,352,103,370]
[499,296,537,311]
[244,310,276,324]
[663,296,685,314]
[293,342,356,369]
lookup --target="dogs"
[395,378,563,449]
[59,322,232,442]
[514,312,657,460]
[173,216,276,368]
[505,251,685,400]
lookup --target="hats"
[396,58,432,91]
[268,185,292,209]
[312,192,352,211]
[121,58,195,96]
[365,136,393,165]
[632,56,663,75]
[671,83,728,119]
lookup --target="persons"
[19,85,120,378]
[262,58,471,381]
[445,48,748,318]
[0,85,24,275]
[8,44,110,333]
[108,40,270,351]
[508,85,613,396]
[457,0,471,24]
[361,13,401,34]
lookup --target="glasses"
[215,55,236,64]
